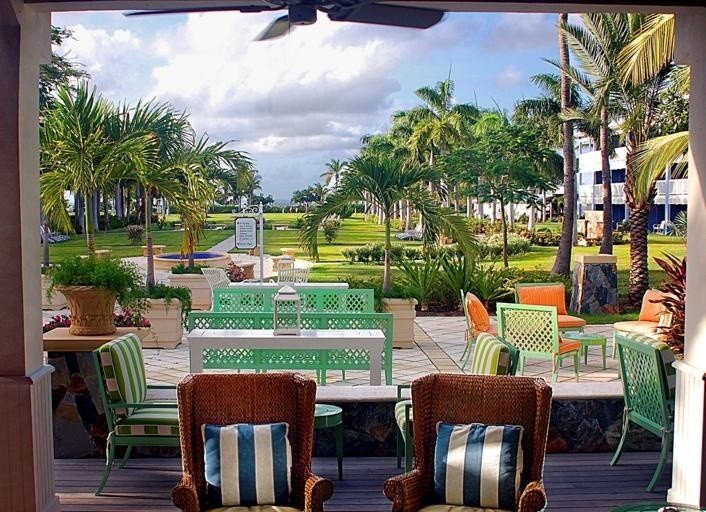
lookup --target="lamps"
[611,330,676,492]
[92,332,178,496]
[200,268,231,312]
[170,373,334,512]
[382,374,551,512]
[269,261,309,308]
[459,281,679,382]
[394,332,521,472]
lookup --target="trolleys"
[122,0,445,42]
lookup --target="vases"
[41,312,153,334]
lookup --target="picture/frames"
[227,282,349,312]
[187,329,386,387]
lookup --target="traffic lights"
[200,422,292,507]
[433,421,526,510]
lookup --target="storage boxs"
[282,249,295,261]
[187,311,392,386]
[213,286,375,384]
[96,250,109,259]
[141,246,165,257]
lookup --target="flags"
[116,326,151,342]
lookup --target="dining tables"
[46,253,148,336]
[167,132,261,312]
[41,214,68,311]
[113,97,215,350]
[298,152,482,349]
